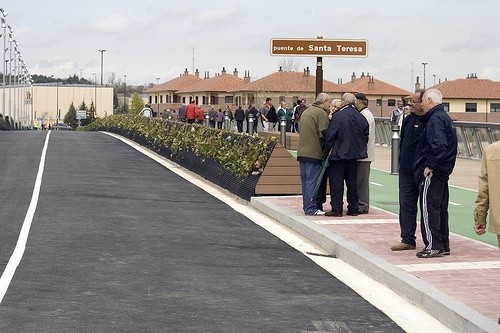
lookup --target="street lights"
[51,74,58,124]
[422,62,428,89]
[156,78,160,84]
[92,72,97,115]
[98,49,106,85]
[124,74,126,112]
[0,8,35,130]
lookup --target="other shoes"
[357,210,368,214]
[346,211,357,216]
[317,204,323,211]
[325,211,342,217]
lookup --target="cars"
[51,122,71,130]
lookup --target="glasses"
[410,98,421,103]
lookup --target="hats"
[355,93,366,100]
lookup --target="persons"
[389,89,458,258]
[474,138,500,325]
[139,92,375,217]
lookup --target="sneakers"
[307,209,326,215]
[444,252,450,255]
[417,249,445,257]
[391,242,416,250]
